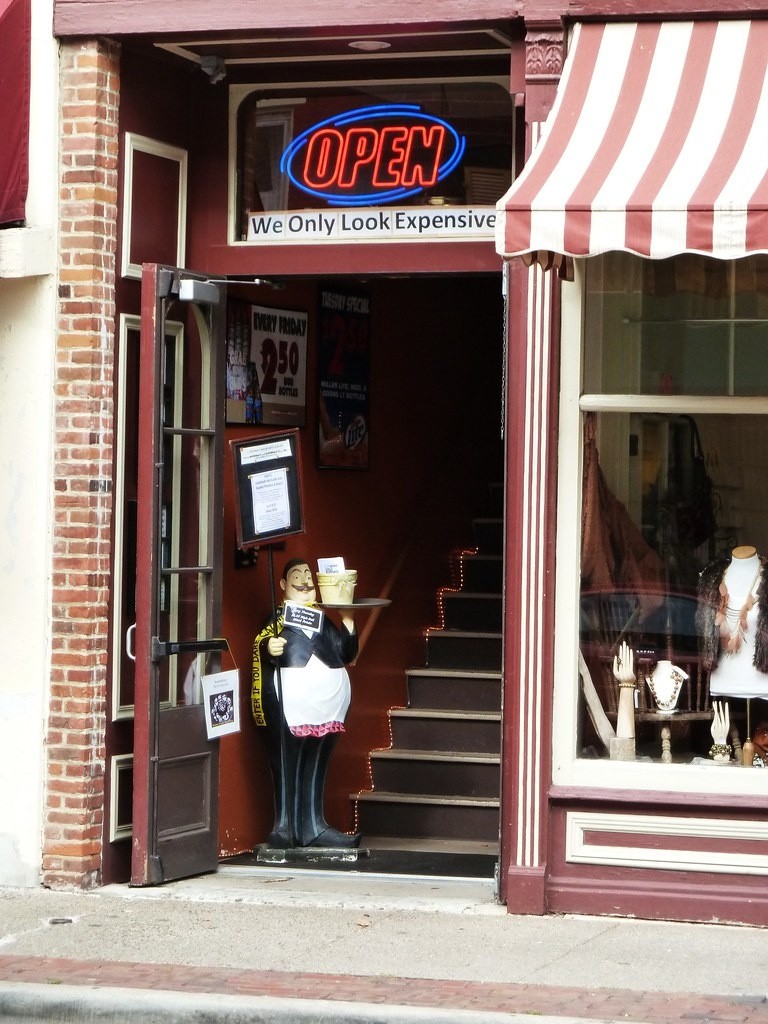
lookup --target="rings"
[616,657,622,664]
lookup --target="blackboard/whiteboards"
[228,427,307,550]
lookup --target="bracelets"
[709,743,733,756]
[618,684,637,688]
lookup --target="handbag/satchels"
[752,720,768,760]
[640,414,718,552]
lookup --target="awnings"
[493,16,768,258]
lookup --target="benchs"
[580,588,768,769]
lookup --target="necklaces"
[712,561,763,655]
[649,664,679,706]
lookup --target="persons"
[578,641,636,757]
[711,701,731,761]
[261,558,362,852]
[693,544,768,705]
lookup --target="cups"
[315,570,357,604]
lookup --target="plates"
[320,598,392,608]
